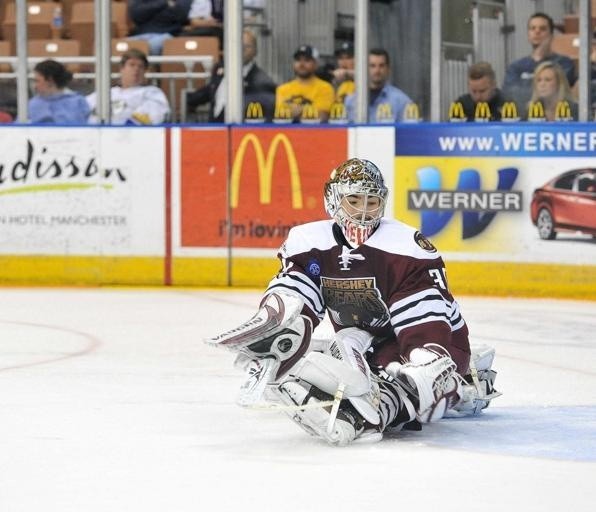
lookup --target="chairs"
[0,1,222,120]
[550,1,596,106]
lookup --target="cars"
[531,168,596,241]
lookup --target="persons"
[274,39,419,124]
[123,1,224,55]
[203,157,497,449]
[85,48,170,124]
[12,59,90,125]
[186,28,276,124]
[450,13,596,121]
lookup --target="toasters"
[51,7,63,27]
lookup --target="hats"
[294,43,320,62]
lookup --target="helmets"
[324,157,388,245]
